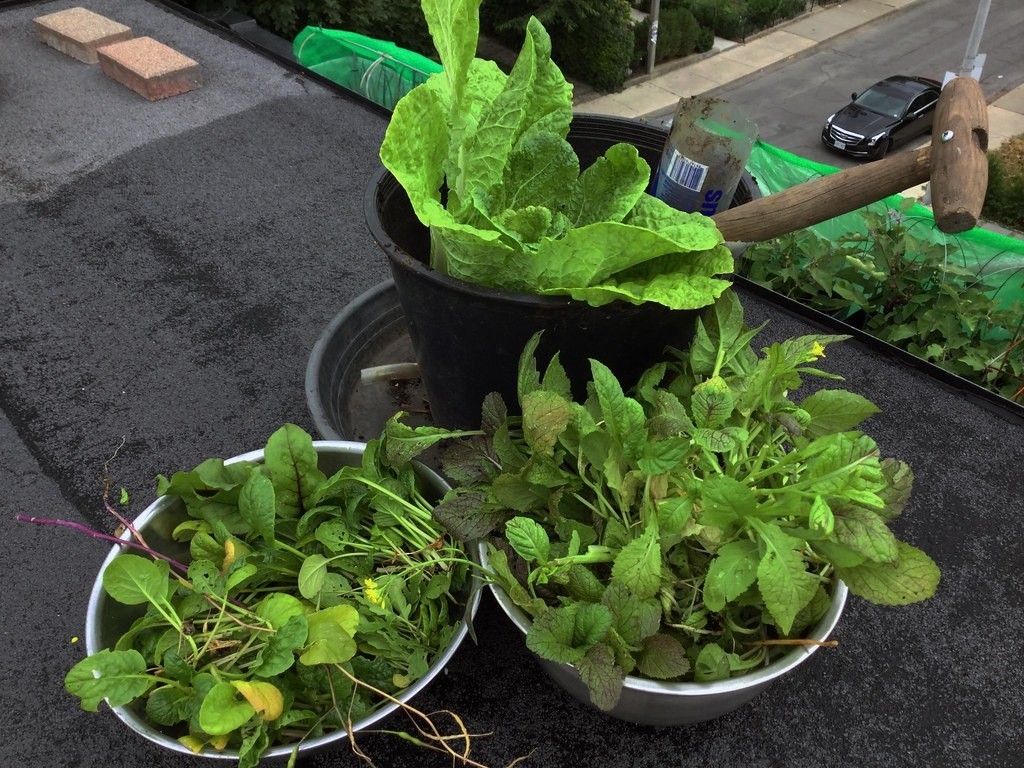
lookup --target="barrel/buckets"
[364,111,763,428]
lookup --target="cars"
[822,74,943,163]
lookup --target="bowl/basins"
[485,578,851,725]
[305,278,437,441]
[86,442,482,760]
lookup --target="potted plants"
[365,0,765,432]
[308,54,416,110]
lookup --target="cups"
[656,97,759,217]
